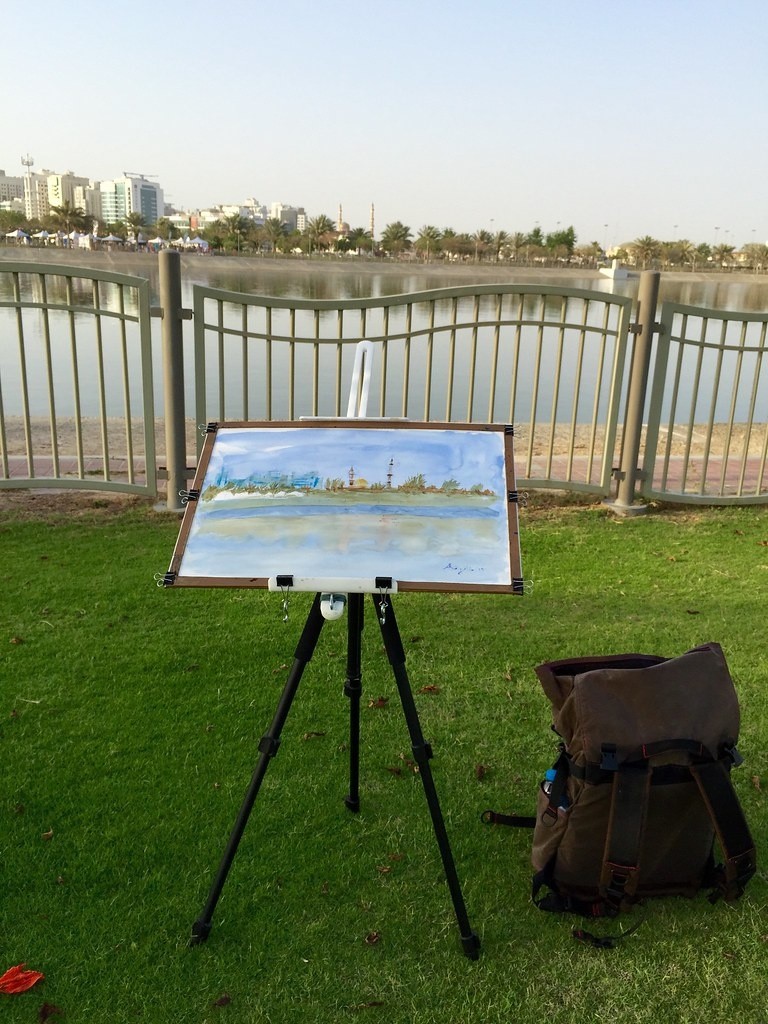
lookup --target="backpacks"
[525,642,753,900]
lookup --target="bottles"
[542,769,564,808]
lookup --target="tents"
[23,230,209,256]
[5,228,29,245]
